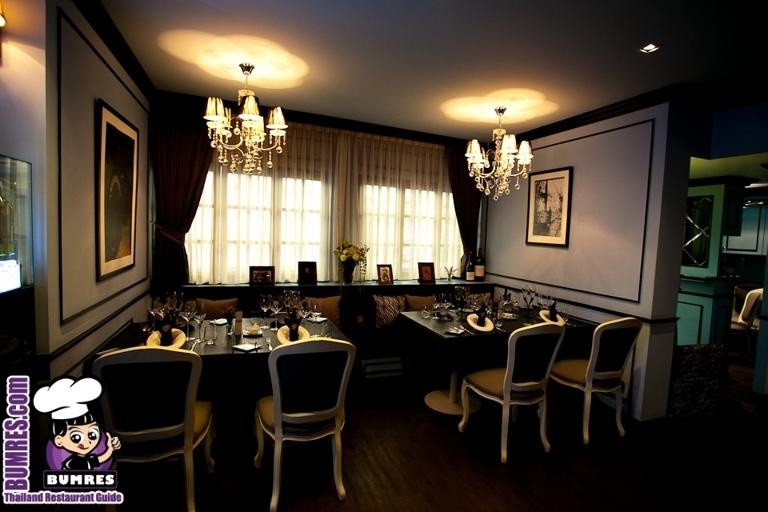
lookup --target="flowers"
[331,239,370,287]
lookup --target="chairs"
[91,346,214,511]
[457,322,566,465]
[550,317,642,445]
[254,338,355,511]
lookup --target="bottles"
[225,310,242,336]
[466,246,484,282]
[502,288,519,312]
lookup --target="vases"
[342,261,355,284]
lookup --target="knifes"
[459,324,474,336]
[190,341,197,351]
[265,336,272,350]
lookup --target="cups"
[420,285,503,328]
[536,289,569,322]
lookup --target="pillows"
[372,294,405,327]
[195,297,237,319]
[299,295,342,329]
[406,295,436,311]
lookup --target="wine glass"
[148,290,217,343]
[257,290,322,330]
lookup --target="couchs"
[161,284,495,400]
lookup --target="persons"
[381,266,389,282]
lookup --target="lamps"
[0,0,8,66]
[203,63,289,177]
[464,106,533,202]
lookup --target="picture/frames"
[525,165,573,248]
[94,97,140,281]
[418,262,435,283]
[250,266,274,286]
[377,264,394,284]
